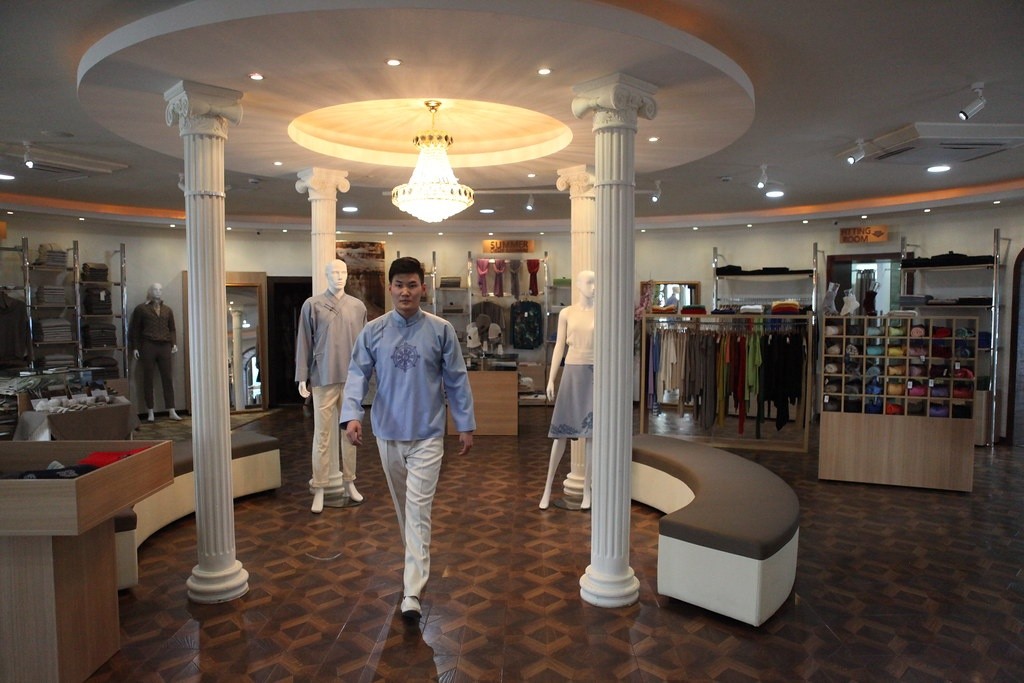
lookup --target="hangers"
[647,296,813,344]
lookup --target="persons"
[539,270,595,509]
[129,282,183,422]
[665,286,680,306]
[294,259,369,513]
[338,256,477,621]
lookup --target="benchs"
[630,433,799,626]
[114,432,282,592]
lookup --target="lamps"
[847,139,864,165]
[526,194,534,210]
[957,82,985,120]
[391,100,473,223]
[650,180,662,203]
[177,173,185,191]
[23,141,34,167]
[756,165,767,189]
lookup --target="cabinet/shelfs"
[710,242,826,425]
[0,236,129,379]
[817,316,980,494]
[396,250,572,365]
[899,227,1012,447]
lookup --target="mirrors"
[183,270,267,416]
[638,280,701,410]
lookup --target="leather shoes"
[399,595,423,619]
